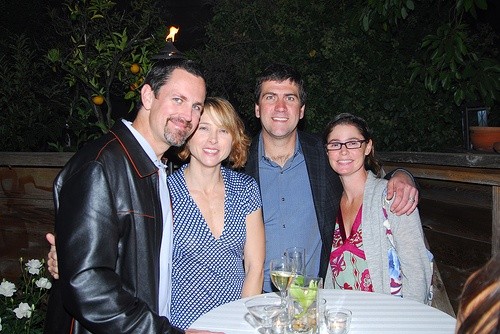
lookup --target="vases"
[470,125,500,152]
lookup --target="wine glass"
[270,258,296,303]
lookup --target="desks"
[186,289,458,334]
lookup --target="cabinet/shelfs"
[377,162,500,303]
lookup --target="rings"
[409,196,415,203]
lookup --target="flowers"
[0,257,54,334]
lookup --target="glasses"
[324,139,367,151]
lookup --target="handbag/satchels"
[382,187,434,307]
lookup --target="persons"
[47,97,265,329]
[322,112,432,304]
[229,63,419,294]
[46,58,224,334]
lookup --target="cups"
[244,247,352,334]
